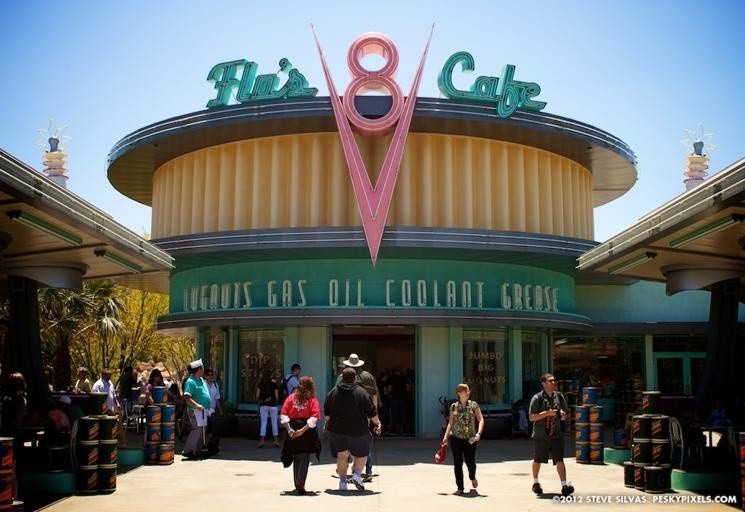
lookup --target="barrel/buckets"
[145,386,176,465]
[558,378,604,465]
[75,392,118,492]
[0,436,15,505]
[616,379,671,494]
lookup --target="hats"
[190,358,204,368]
[342,352,365,368]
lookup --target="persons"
[442,384,485,495]
[256,353,410,494]
[74,358,224,459]
[711,400,736,426]
[0,371,28,438]
[529,373,574,496]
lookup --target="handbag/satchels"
[434,443,446,464]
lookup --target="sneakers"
[561,485,574,496]
[531,482,542,493]
[351,474,365,491]
[338,480,347,491]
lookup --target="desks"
[21,426,45,446]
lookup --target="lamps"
[607,251,657,276]
[669,214,741,250]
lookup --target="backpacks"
[279,375,296,405]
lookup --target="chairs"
[125,399,147,433]
[511,399,533,440]
[47,418,79,472]
